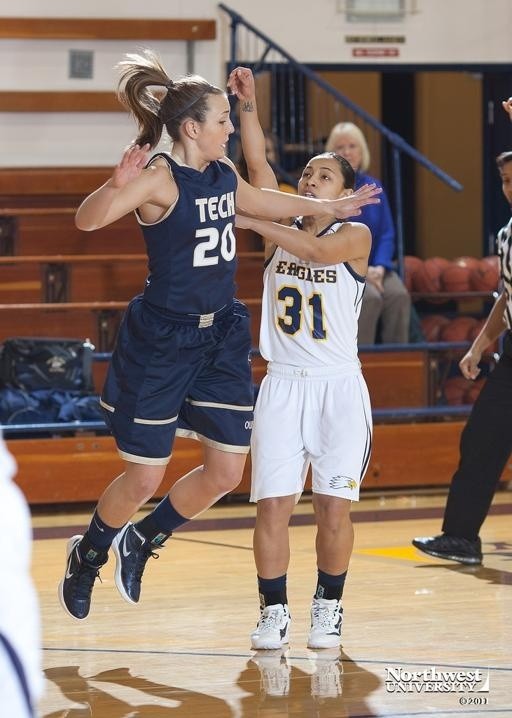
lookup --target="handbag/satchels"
[0,338,113,439]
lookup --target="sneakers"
[57,534,109,621]
[411,533,482,564]
[307,598,344,648]
[110,521,165,606]
[250,603,291,650]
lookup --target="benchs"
[1,163,511,505]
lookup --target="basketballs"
[399,255,501,408]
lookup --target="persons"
[2,427,42,717]
[235,128,298,220]
[223,61,376,649]
[61,50,385,619]
[47,661,237,718]
[240,655,385,717]
[322,122,413,343]
[412,94,512,566]
